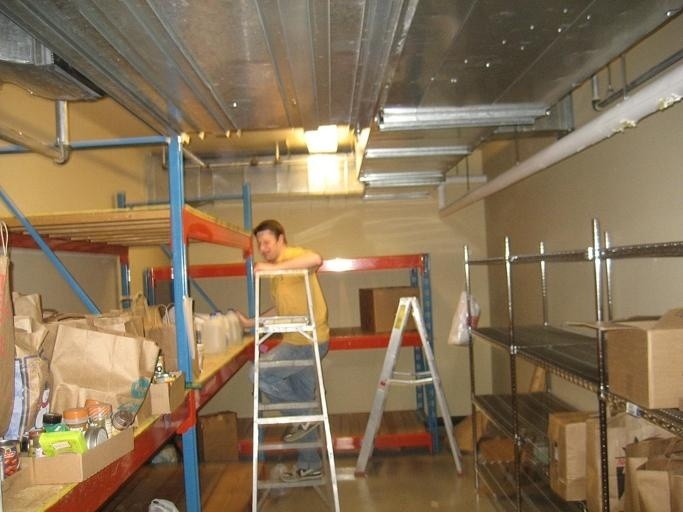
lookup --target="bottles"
[156,354,165,377]
[0,399,135,482]
[202,308,244,352]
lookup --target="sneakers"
[283,420,324,442]
[279,463,326,482]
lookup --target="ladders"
[355,296,464,477]
[252,268,341,512]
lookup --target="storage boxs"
[547,307,683,512]
[196,412,240,466]
[358,285,419,333]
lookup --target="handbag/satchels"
[447,290,481,346]
[623,436,683,512]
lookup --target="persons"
[235,219,331,482]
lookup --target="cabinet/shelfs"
[145,249,439,457]
[0,131,265,512]
[463,217,683,512]
